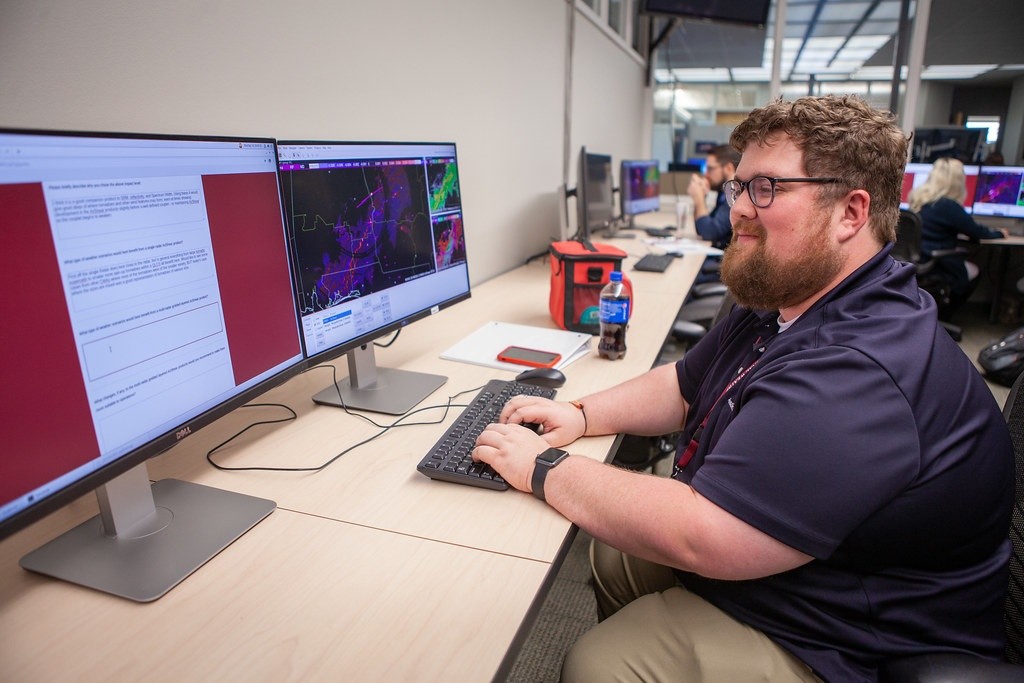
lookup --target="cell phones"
[497,347,562,369]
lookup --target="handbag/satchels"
[549,238,632,331]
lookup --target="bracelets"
[568,400,588,436]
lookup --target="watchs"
[530,446,571,504]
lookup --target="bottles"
[598,271,631,360]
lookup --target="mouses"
[667,253,684,258]
[666,226,677,231]
[516,368,567,388]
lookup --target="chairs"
[876,370,1024,683]
[887,209,970,341]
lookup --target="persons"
[906,156,1010,325]
[687,144,742,302]
[471,92,1016,683]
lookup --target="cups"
[676,202,689,238]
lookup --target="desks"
[957,232,1024,325]
[0,192,717,683]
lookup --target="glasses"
[722,176,847,209]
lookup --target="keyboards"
[416,379,557,492]
[644,228,673,237]
[633,254,672,273]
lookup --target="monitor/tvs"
[570,144,662,242]
[640,0,771,31]
[0,125,475,601]
[899,162,1024,220]
